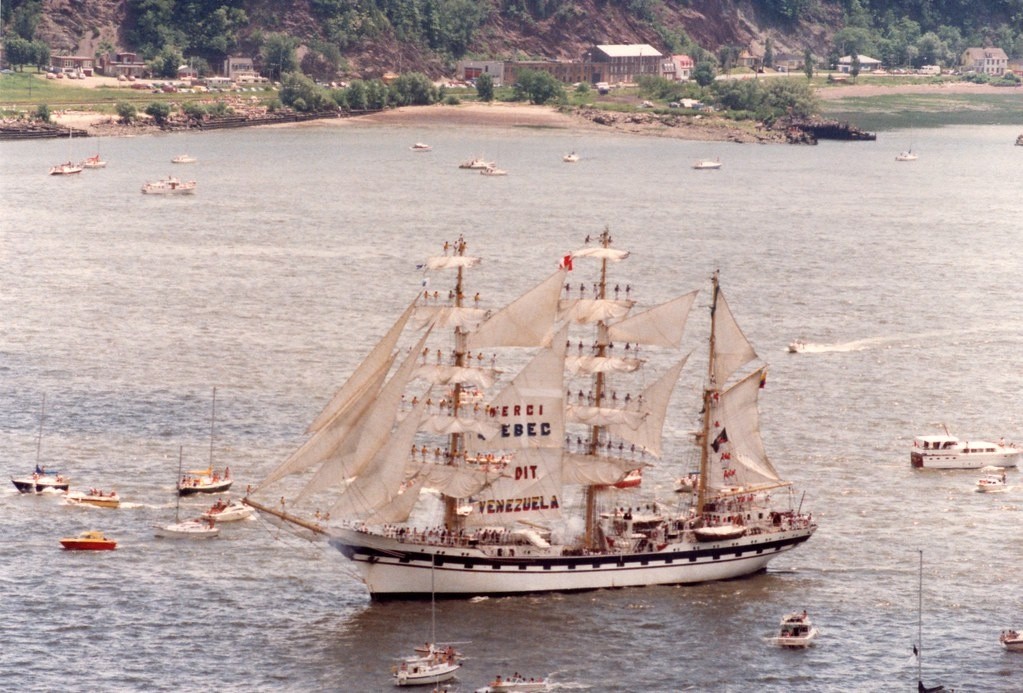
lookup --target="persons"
[433,689,447,693]
[89,487,116,498]
[181,464,229,486]
[998,436,1017,448]
[400,233,512,471]
[679,375,739,486]
[568,150,574,158]
[999,629,1018,646]
[246,485,513,557]
[32,465,62,482]
[1001,472,1007,484]
[53,153,99,171]
[467,159,496,174]
[564,232,647,462]
[85,533,107,541]
[604,493,813,554]
[782,610,807,637]
[391,641,454,675]
[496,672,543,684]
[474,690,488,693]
[202,496,230,531]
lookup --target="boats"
[407,141,724,178]
[910,433,1022,472]
[1000,629,1023,651]
[489,681,547,693]
[897,149,917,162]
[1014,135,1023,146]
[976,465,1008,493]
[772,611,818,649]
[138,153,196,198]
[59,531,117,551]
[788,337,809,354]
[62,492,120,507]
[201,503,254,522]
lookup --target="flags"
[419,277,431,288]
[556,254,573,271]
[758,370,767,389]
[710,427,728,453]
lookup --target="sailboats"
[149,444,221,541]
[908,548,944,693]
[176,386,232,497]
[48,125,109,176]
[242,227,825,604]
[390,554,465,686]
[12,392,70,493]
